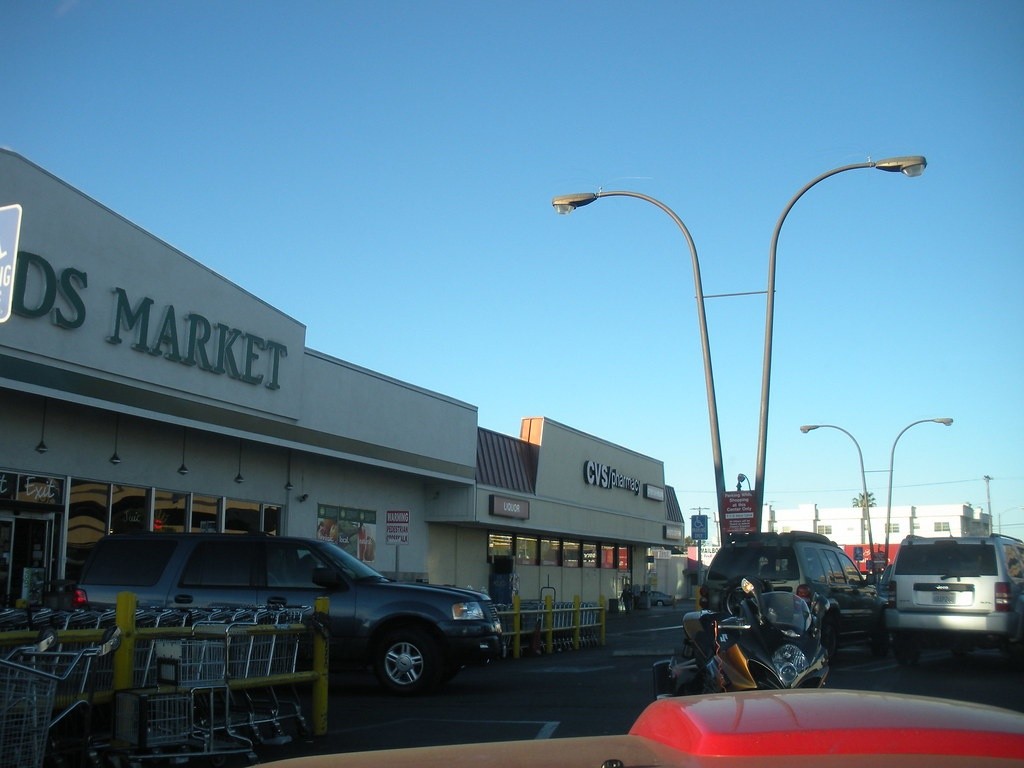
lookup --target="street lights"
[799,416,954,545]
[551,154,928,545]
[998,506,1024,533]
[983,475,994,536]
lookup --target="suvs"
[73,532,507,695]
[699,531,889,656]
[880,533,1024,668]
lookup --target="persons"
[620,584,633,616]
[299,554,316,574]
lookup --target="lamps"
[177,426,189,475]
[34,397,48,454]
[286,449,293,490]
[234,439,245,483]
[109,413,121,464]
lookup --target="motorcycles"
[671,576,840,695]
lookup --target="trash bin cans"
[640,591,651,608]
[608,599,618,613]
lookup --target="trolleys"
[0,607,316,768]
[495,603,604,656]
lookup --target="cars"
[878,564,892,605]
[620,584,675,607]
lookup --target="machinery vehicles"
[812,504,820,534]
[765,503,777,533]
[910,506,917,535]
